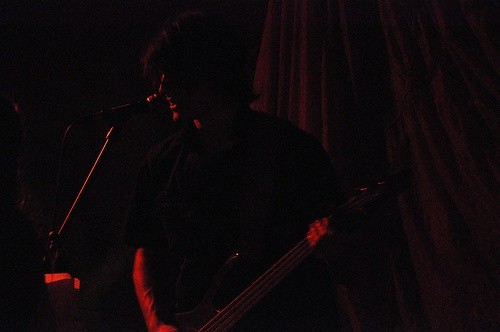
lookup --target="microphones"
[70,94,164,132]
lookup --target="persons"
[1,95,45,332]
[119,11,358,332]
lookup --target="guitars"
[80,167,414,332]
